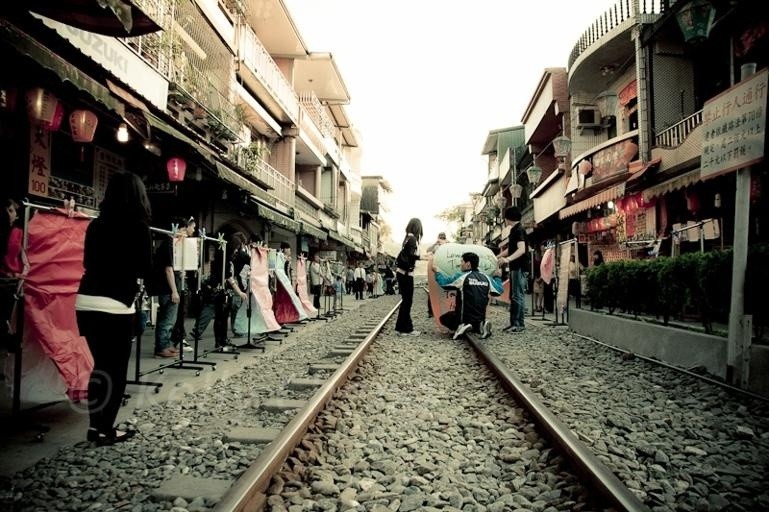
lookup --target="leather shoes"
[154,347,181,357]
[87,427,135,448]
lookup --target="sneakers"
[396,330,421,337]
[536,307,543,312]
[190,331,205,340]
[453,323,473,340]
[503,325,525,333]
[356,298,365,300]
[215,339,236,347]
[479,320,493,339]
[173,339,194,352]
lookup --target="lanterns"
[165,157,189,182]
[68,110,99,146]
[570,188,656,238]
[30,84,64,135]
[679,192,705,212]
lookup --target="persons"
[146,209,397,362]
[75,170,157,446]
[394,216,427,338]
[530,245,603,316]
[497,207,527,332]
[0,196,30,384]
[424,230,454,320]
[433,250,504,342]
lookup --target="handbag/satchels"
[324,285,336,296]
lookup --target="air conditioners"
[576,106,601,125]
